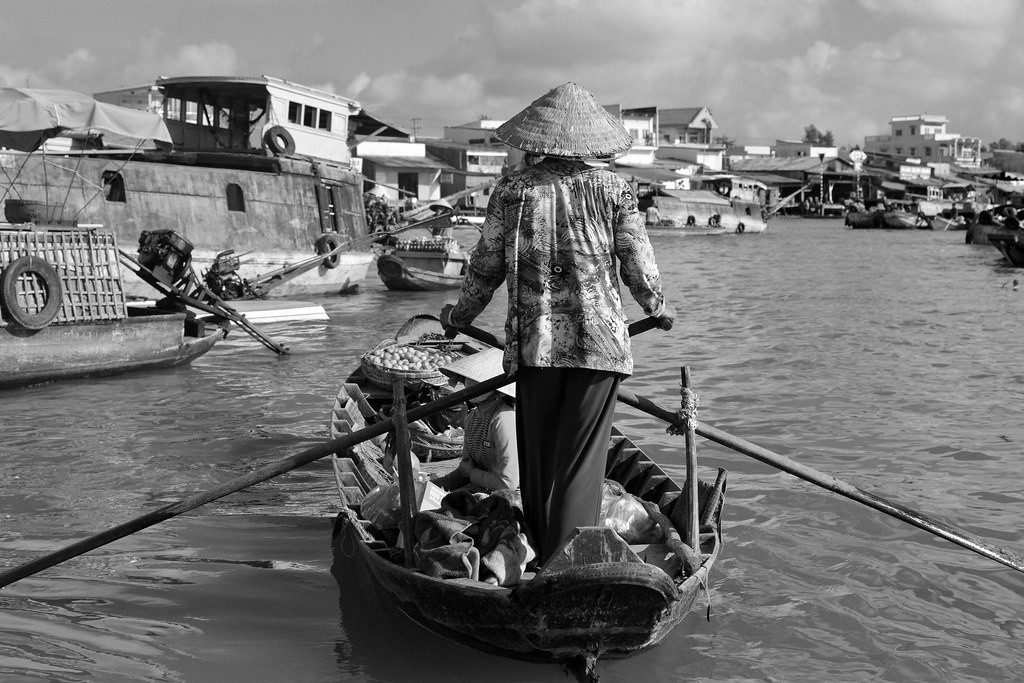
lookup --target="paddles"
[1,316,673,588]
[445,323,1020,573]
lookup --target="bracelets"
[448,308,456,326]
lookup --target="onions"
[369,345,452,370]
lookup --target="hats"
[438,347,516,398]
[494,82,634,161]
[430,198,455,217]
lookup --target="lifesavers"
[0,255,64,330]
[688,215,696,225]
[319,235,340,268]
[265,125,295,155]
[709,217,716,226]
[738,223,745,233]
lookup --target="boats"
[0,86,292,387]
[331,312,730,683]
[637,175,770,234]
[125,249,330,326]
[0,72,378,301]
[844,198,1024,269]
[376,236,469,293]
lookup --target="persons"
[439,81,676,567]
[427,347,519,493]
[645,203,660,226]
[1001,206,1019,230]
[430,197,453,236]
